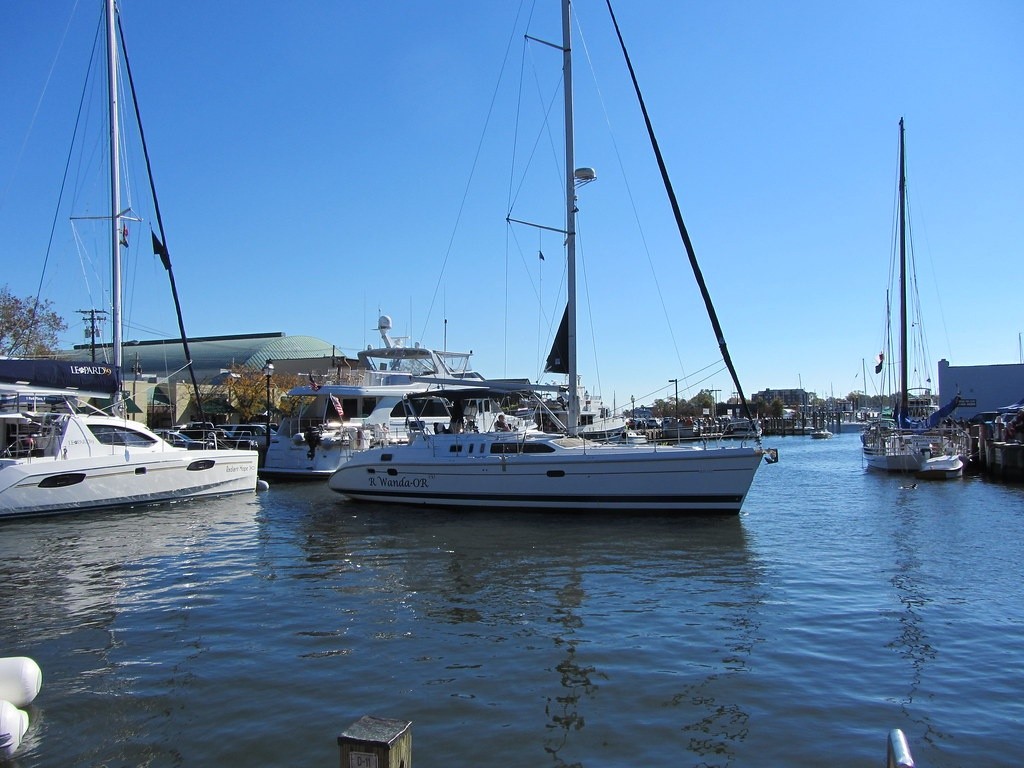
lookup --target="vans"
[218,424,280,448]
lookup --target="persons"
[943,409,1024,442]
[495,414,512,432]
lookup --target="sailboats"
[861,112,975,471]
[327,0,780,516]
[0,0,261,516]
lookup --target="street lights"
[266,361,274,448]
[668,379,681,444]
[631,394,635,429]
[709,390,723,418]
[732,391,739,407]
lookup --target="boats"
[259,304,649,479]
[810,429,833,438]
[913,453,964,479]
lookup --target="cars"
[636,417,648,429]
[661,416,712,427]
[721,415,731,421]
[626,417,635,429]
[648,418,662,427]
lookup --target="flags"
[308,372,320,391]
[876,362,882,374]
[152,231,171,270]
[329,395,344,416]
